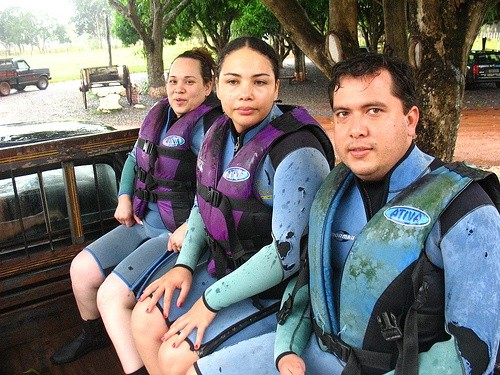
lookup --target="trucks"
[0,58,53,96]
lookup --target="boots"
[50,316,110,365]
[126,366,150,375]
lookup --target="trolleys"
[79,65,133,109]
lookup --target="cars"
[360,46,370,54]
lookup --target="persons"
[51,37,499,375]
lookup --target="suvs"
[466,50,500,89]
